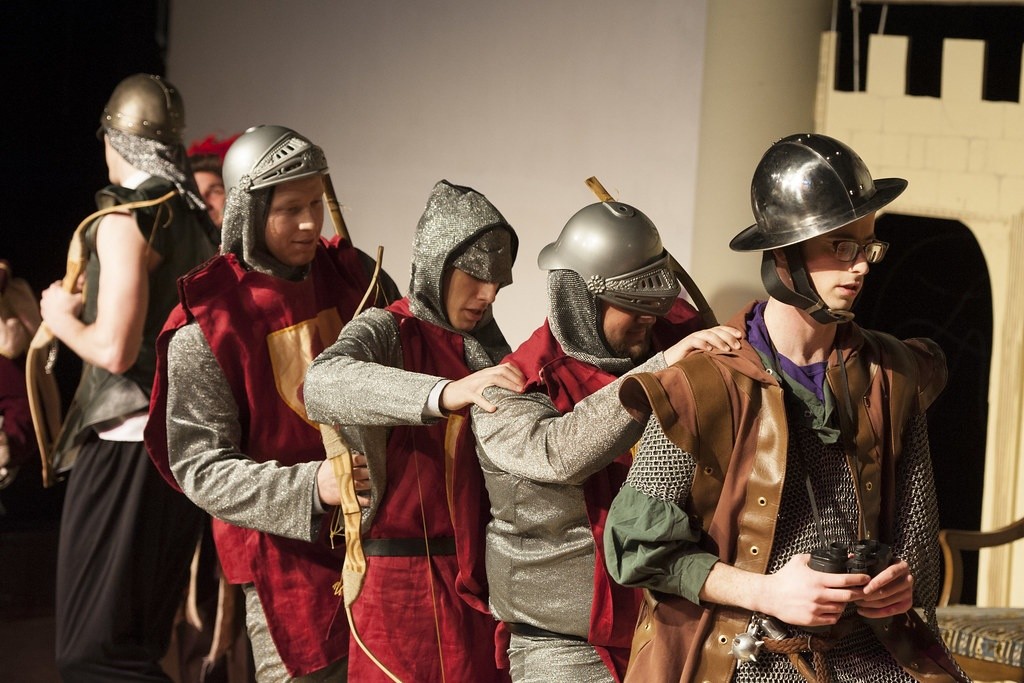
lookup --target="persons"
[303,180,526,683]
[471,202,743,683]
[604,134,972,683]
[0,258,59,613]
[186,134,245,250]
[25,75,222,683]
[143,123,402,683]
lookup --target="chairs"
[918,517,1024,683]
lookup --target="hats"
[450,227,517,286]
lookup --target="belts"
[362,537,456,557]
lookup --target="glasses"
[806,237,890,264]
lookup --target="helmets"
[729,133,909,253]
[100,72,187,149]
[537,201,681,316]
[222,124,329,198]
